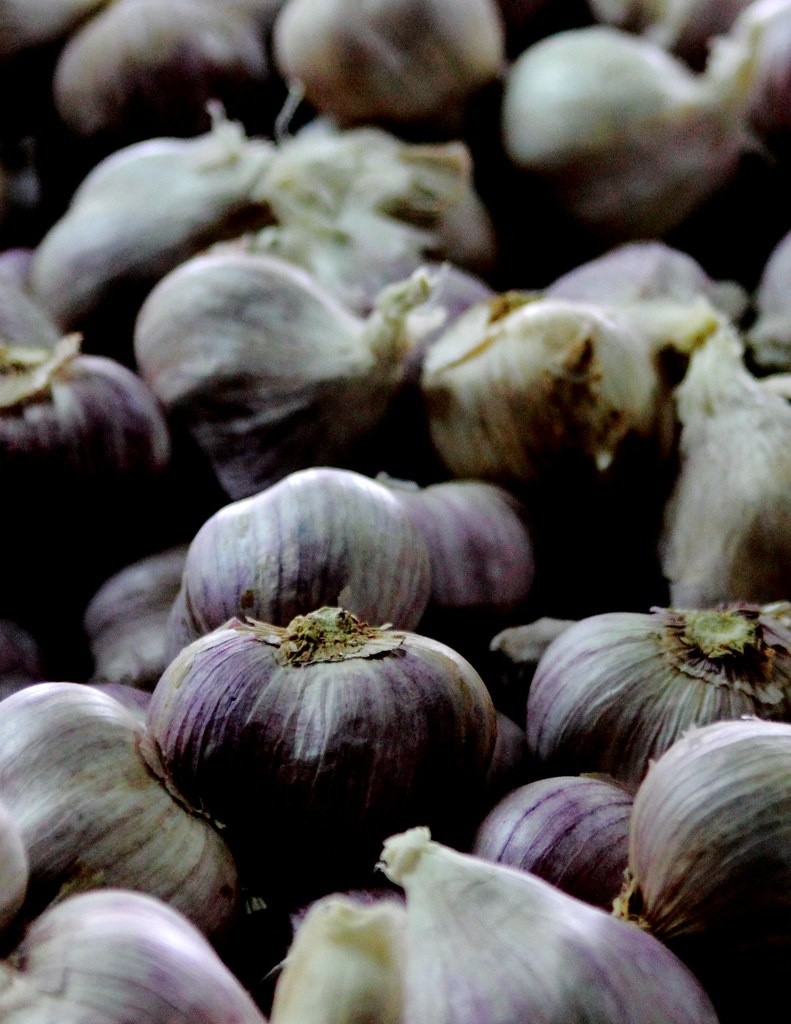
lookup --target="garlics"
[0,0,791,1024]
